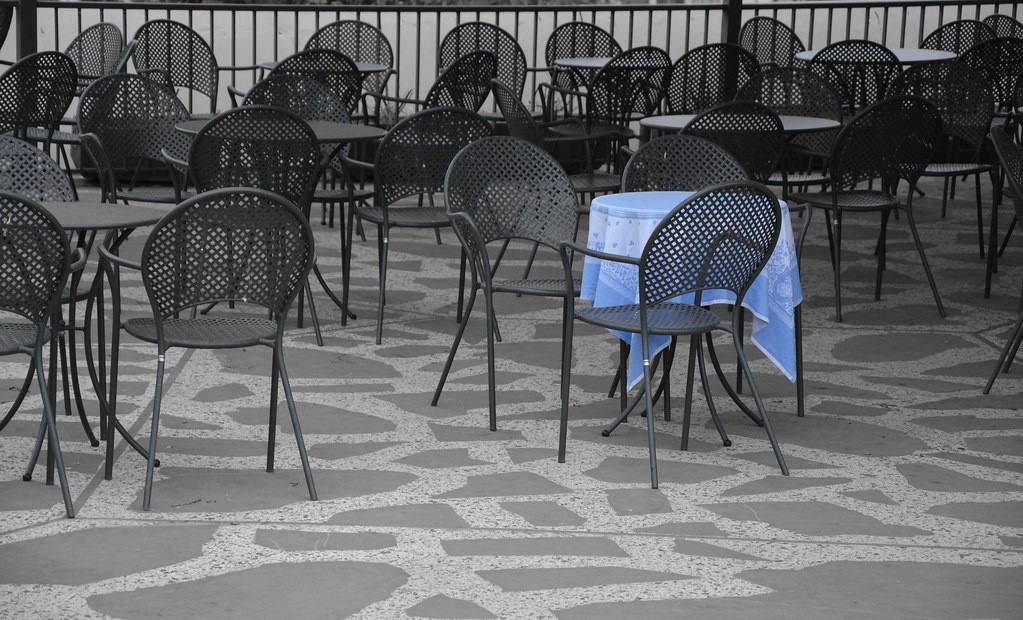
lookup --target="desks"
[639,111,842,190]
[793,45,958,198]
[172,116,386,350]
[259,61,389,187]
[552,57,670,173]
[0,201,185,487]
[579,191,804,453]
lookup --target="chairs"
[0,3,1023,520]
